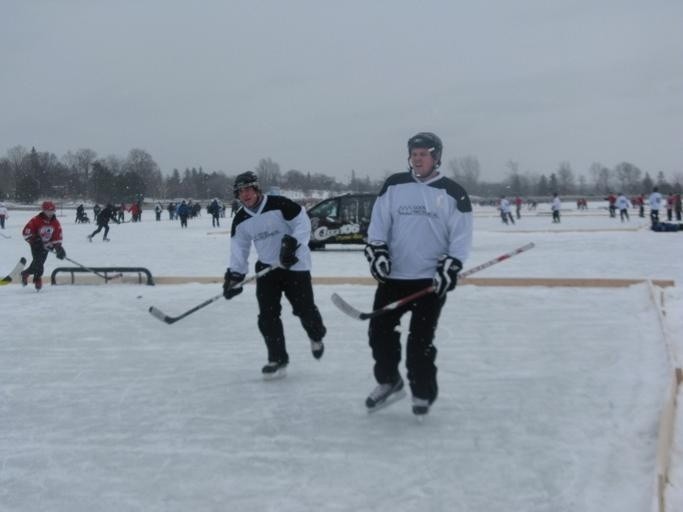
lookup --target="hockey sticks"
[52,250,122,280]
[144,262,280,324]
[331,243,533,321]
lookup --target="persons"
[513,193,522,217]
[604,192,617,218]
[221,170,328,375]
[526,194,539,219]
[648,186,662,229]
[20,202,65,293]
[188,197,203,219]
[118,201,141,223]
[666,190,682,221]
[74,203,90,224]
[549,193,562,224]
[94,203,100,221]
[154,201,160,218]
[576,196,588,211]
[362,131,475,420]
[0,202,8,231]
[166,201,180,220]
[495,195,516,226]
[176,199,191,230]
[610,193,631,223]
[630,190,646,218]
[207,198,222,228]
[527,201,540,212]
[87,203,121,243]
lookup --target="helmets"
[42,201,57,212]
[233,172,260,199]
[408,133,443,179]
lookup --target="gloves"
[222,268,245,300]
[433,252,463,299]
[279,234,299,268]
[364,244,391,284]
[42,241,52,252]
[56,246,66,260]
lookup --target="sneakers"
[88,235,93,241]
[412,397,430,415]
[21,270,30,286]
[262,361,285,373]
[103,238,110,241]
[310,339,325,359]
[366,372,404,406]
[33,276,42,289]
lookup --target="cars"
[307,193,379,250]
